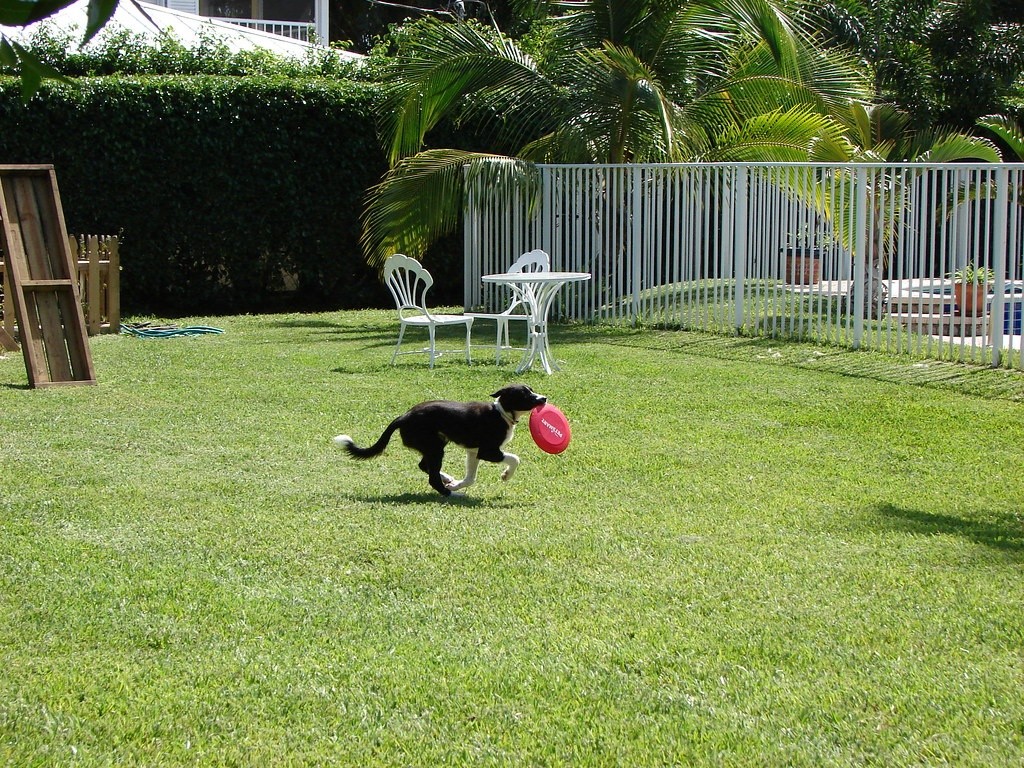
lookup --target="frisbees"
[529,404,571,454]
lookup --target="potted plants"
[945,257,995,318]
[779,217,840,285]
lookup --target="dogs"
[332,383,547,499]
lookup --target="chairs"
[464,247,551,366]
[379,253,474,370]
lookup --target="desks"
[481,272,593,376]
